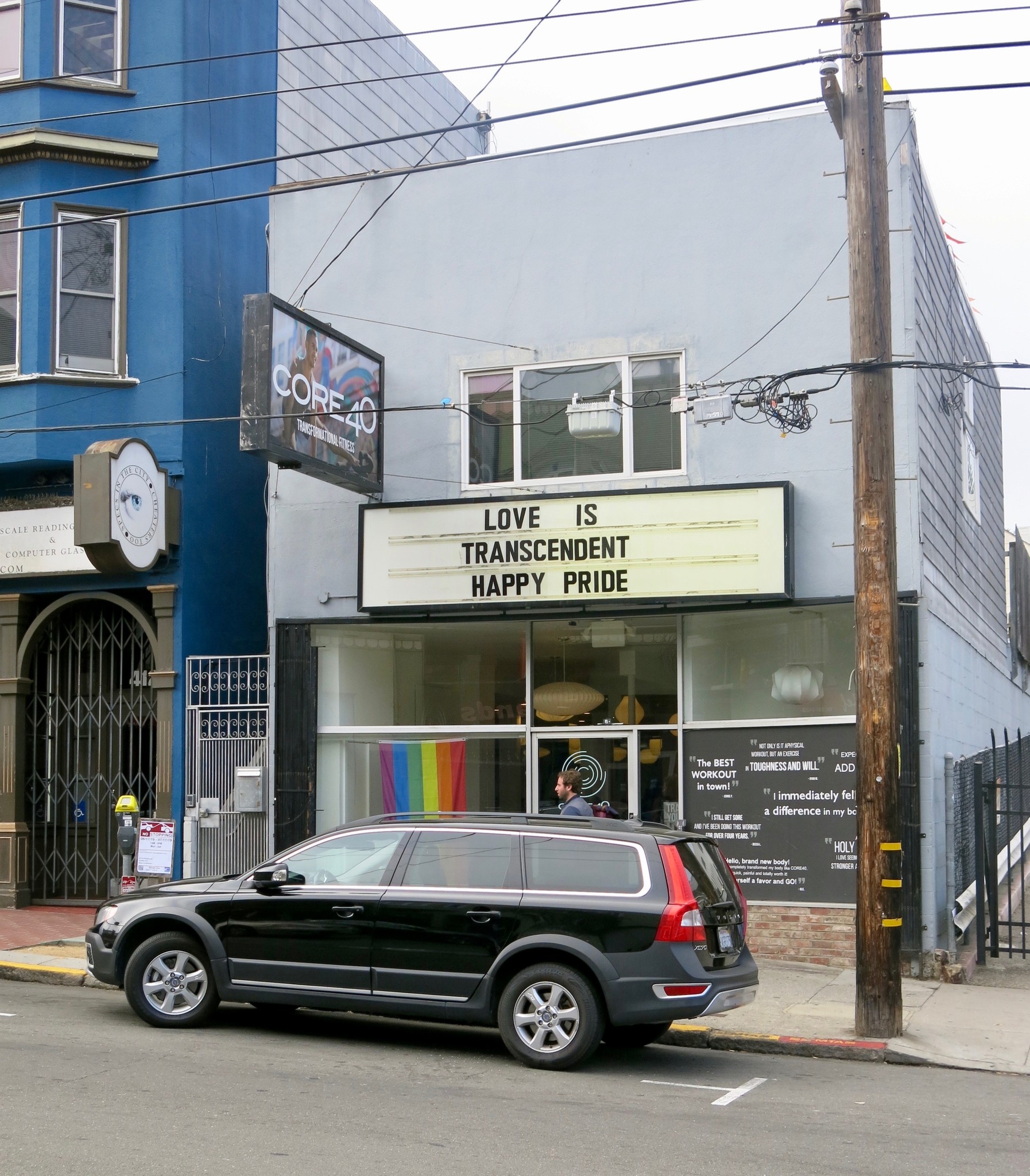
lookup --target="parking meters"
[114,796,140,895]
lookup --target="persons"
[555,769,594,817]
[280,328,361,469]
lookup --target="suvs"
[84,808,759,1072]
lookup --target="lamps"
[520,636,678,764]
